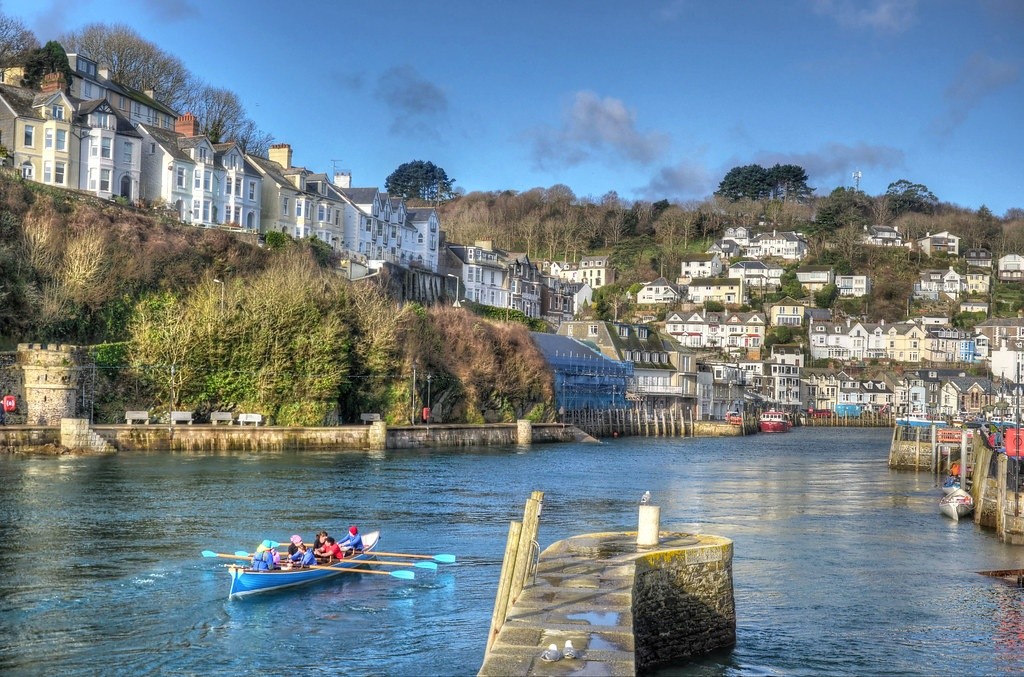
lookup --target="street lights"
[447,274,460,308]
[69,115,93,190]
[904,377,910,424]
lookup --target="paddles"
[201,548,417,581]
[260,538,457,567]
[233,550,438,572]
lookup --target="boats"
[960,416,994,428]
[939,489,974,523]
[942,474,973,494]
[895,412,947,428]
[990,417,1024,429]
[936,416,963,428]
[228,531,381,600]
[759,408,788,433]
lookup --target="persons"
[290,545,318,570]
[988,432,996,447]
[336,526,364,557]
[314,537,343,559]
[994,428,1002,449]
[252,539,280,571]
[313,530,328,557]
[950,463,959,478]
[287,535,306,560]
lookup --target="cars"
[731,417,742,425]
[725,412,739,421]
[812,409,836,418]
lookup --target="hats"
[290,535,302,544]
[257,540,280,552]
[349,526,358,535]
